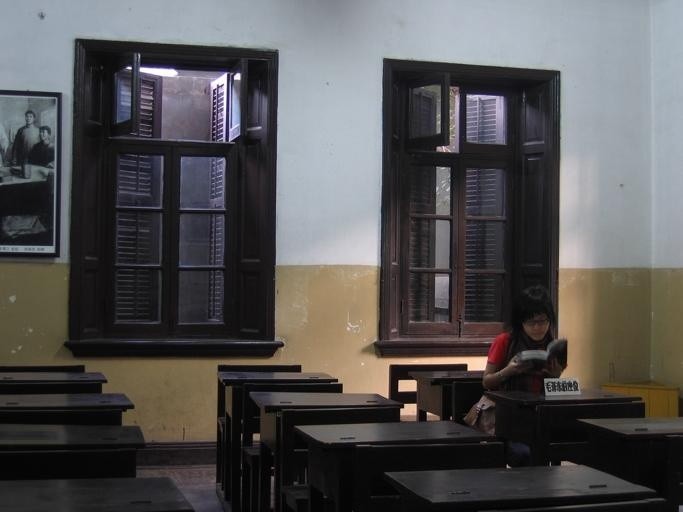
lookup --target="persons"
[465,285,567,470]
[28,124,55,170]
[11,107,40,163]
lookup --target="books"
[516,336,568,379]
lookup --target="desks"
[384,463,667,511]
[1,478,195,511]
[295,421,505,512]
[575,417,682,511]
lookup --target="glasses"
[524,318,548,327]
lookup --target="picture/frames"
[0,89,64,259]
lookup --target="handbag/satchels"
[463,394,497,436]
